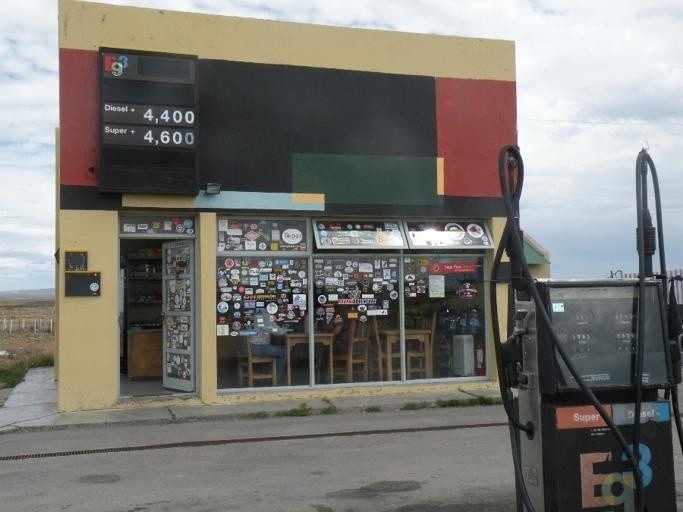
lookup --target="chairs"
[236,310,438,387]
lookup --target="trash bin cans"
[452,334,476,376]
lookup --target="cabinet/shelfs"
[124,245,163,381]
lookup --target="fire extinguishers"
[476,342,485,376]
